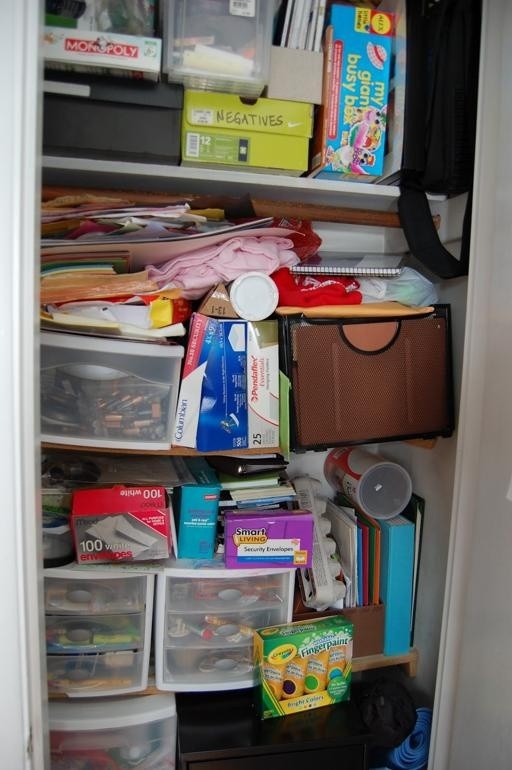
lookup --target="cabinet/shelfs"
[44,567,155,700]
[48,695,179,770]
[179,697,377,770]
[0,1,512,770]
[155,561,297,693]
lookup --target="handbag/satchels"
[398,0,483,279]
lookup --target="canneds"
[323,447,414,521]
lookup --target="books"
[271,0,326,50]
[212,453,299,555]
[289,265,400,279]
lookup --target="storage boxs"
[280,303,454,450]
[261,43,325,105]
[43,23,164,79]
[179,87,312,177]
[293,603,385,659]
[41,331,185,452]
[70,486,171,564]
[44,67,182,166]
[159,0,276,96]
[311,2,396,184]
